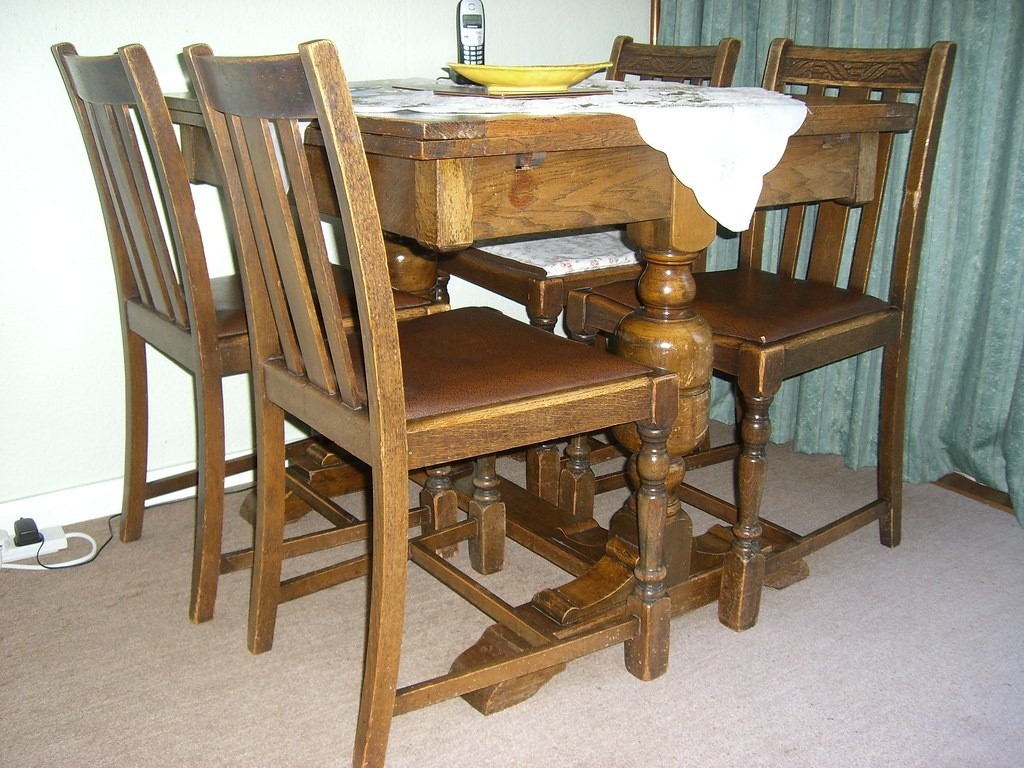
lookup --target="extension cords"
[0,526,67,563]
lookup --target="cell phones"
[456,0,485,67]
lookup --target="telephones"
[449,0,485,85]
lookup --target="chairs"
[183,39,681,768]
[51,42,450,625]
[432,36,747,505]
[557,41,957,631]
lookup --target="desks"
[161,79,918,715]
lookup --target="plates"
[449,62,613,92]
